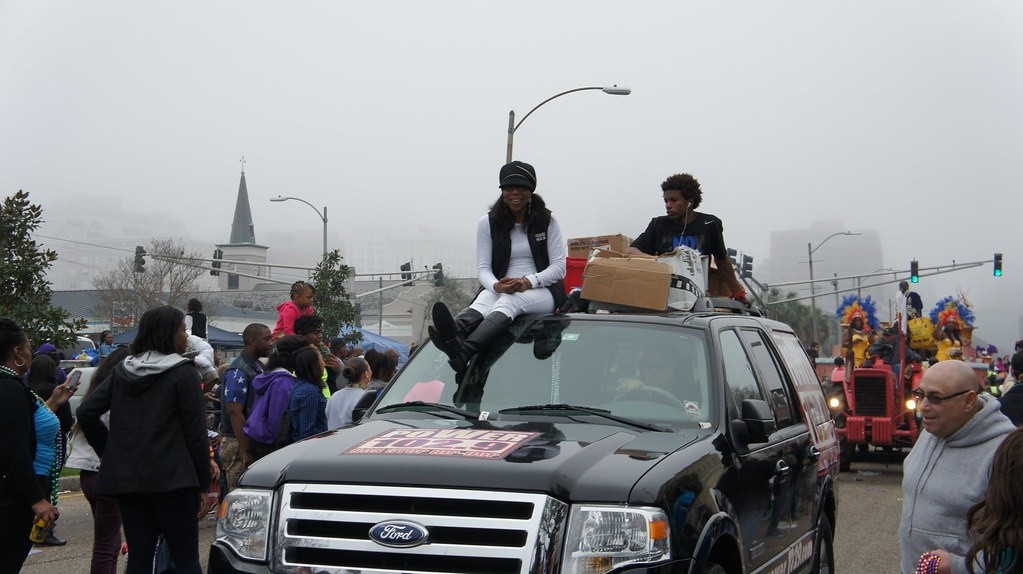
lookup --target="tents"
[337,324,409,370]
[92,326,138,349]
[207,325,244,359]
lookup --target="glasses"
[913,389,971,405]
[504,184,530,191]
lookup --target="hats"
[499,161,537,192]
[883,327,894,335]
[33,343,57,354]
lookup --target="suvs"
[204,300,841,574]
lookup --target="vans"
[42,336,97,358]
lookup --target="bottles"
[319,341,330,362]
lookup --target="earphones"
[687,202,691,208]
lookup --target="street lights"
[506,83,632,164]
[269,194,327,263]
[806,230,864,345]
[857,268,898,299]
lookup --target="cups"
[29,507,58,542]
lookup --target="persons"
[429,161,568,374]
[63,344,124,574]
[185,297,210,341]
[893,280,923,325]
[628,172,752,310]
[99,331,119,356]
[862,328,914,378]
[896,360,1017,574]
[0,317,81,574]
[24,342,76,546]
[616,345,700,407]
[839,315,876,369]
[216,279,401,520]
[931,318,971,363]
[75,305,221,574]
[806,343,820,371]
[964,339,1023,574]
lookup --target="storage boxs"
[562,235,744,311]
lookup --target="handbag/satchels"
[278,409,293,444]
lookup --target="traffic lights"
[133,246,146,273]
[911,260,919,283]
[400,262,413,286]
[432,263,445,287]
[726,248,737,265]
[741,254,753,279]
[992,252,1003,276]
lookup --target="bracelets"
[731,291,746,300]
[534,273,542,288]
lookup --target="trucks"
[825,313,991,473]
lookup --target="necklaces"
[0,366,62,506]
[915,552,941,574]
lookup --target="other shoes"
[36,534,67,545]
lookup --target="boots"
[428,311,511,374]
[433,302,484,355]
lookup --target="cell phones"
[68,371,82,390]
